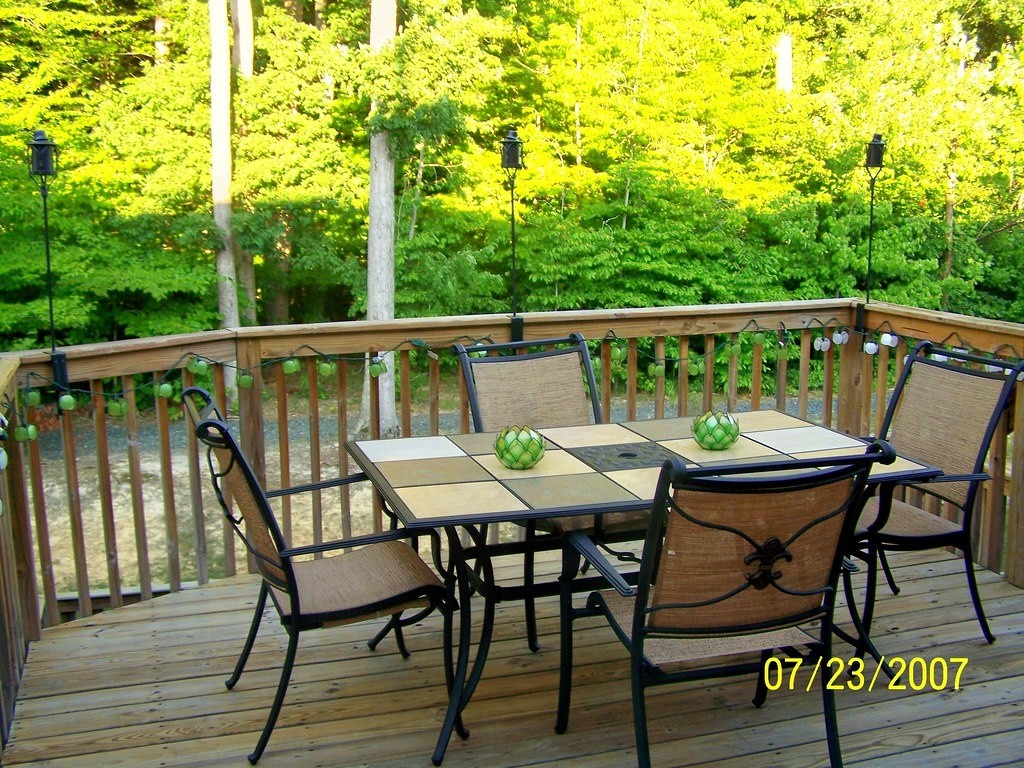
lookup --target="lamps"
[500,130,526,179]
[25,130,57,189]
[863,133,887,180]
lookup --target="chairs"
[181,389,459,767]
[833,340,1024,677]
[552,441,897,768]
[452,333,668,652]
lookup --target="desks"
[343,409,945,768]
